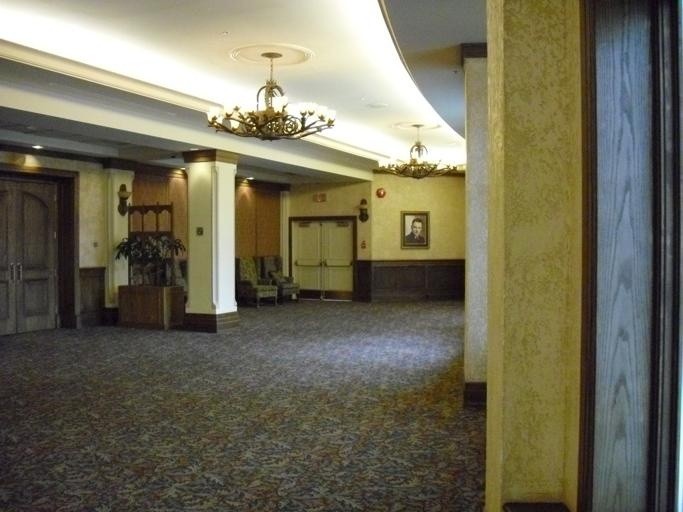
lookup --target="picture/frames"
[400,209,429,249]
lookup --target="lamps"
[207,52,336,143]
[116,182,132,217]
[355,198,370,223]
[372,124,459,180]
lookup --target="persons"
[405,217,425,245]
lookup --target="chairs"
[254,254,301,303]
[236,255,279,310]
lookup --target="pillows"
[268,266,288,284]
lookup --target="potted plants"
[112,234,188,331]
